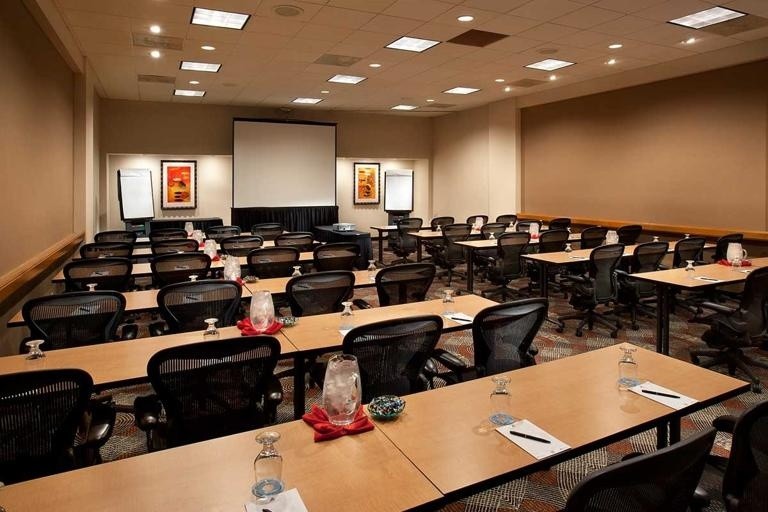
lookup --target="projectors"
[333,223,356,231]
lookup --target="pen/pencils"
[80,307,90,312]
[186,296,198,300]
[510,430,551,444]
[641,390,680,398]
[263,509,272,512]
[451,317,471,321]
[297,285,310,288]
[700,278,718,281]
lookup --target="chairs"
[560,425,717,512]
[621,400,768,511]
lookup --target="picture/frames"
[354,163,381,204]
[161,160,196,210]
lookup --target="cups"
[727,242,747,264]
[185,222,363,425]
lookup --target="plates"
[367,394,406,420]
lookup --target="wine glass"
[488,376,515,425]
[367,259,378,277]
[732,258,741,271]
[618,347,638,390]
[436,222,695,277]
[252,430,283,499]
[443,289,456,317]
[25,340,46,372]
[86,283,98,291]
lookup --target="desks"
[361,342,751,505]
[1,406,444,512]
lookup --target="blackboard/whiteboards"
[115,169,155,221]
[383,167,415,213]
